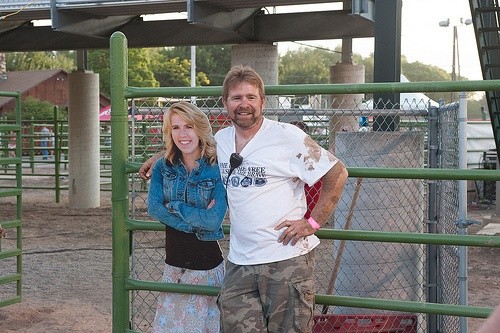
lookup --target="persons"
[289,120,324,222]
[103,127,112,158]
[147,102,229,333]
[10,127,54,161]
[481,107,486,121]
[139,64,348,333]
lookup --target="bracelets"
[307,217,320,230]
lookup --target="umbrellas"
[99,105,143,120]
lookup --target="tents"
[364,74,441,123]
